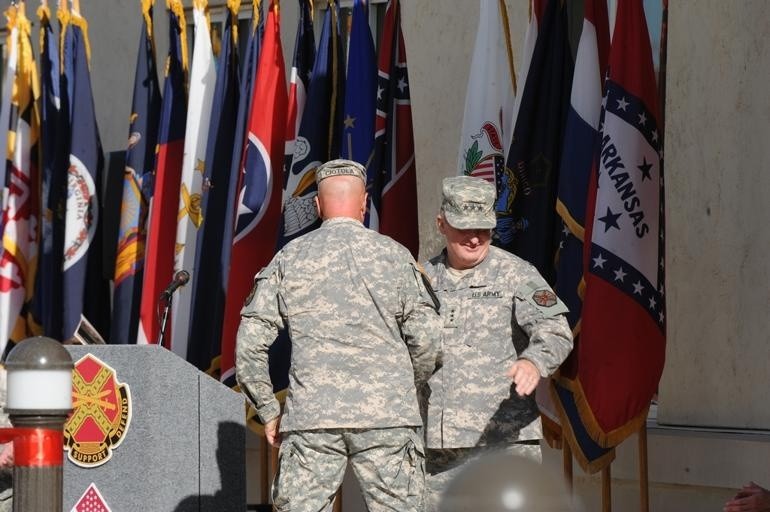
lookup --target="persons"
[418,176,574,512]
[724,482,770,512]
[234,159,444,512]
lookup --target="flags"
[1,0,418,436]
[573,0,661,447]
[457,1,518,246]
[503,2,573,449]
[555,0,625,473]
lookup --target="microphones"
[157,270,190,302]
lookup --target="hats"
[316,158,367,183]
[441,176,498,230]
